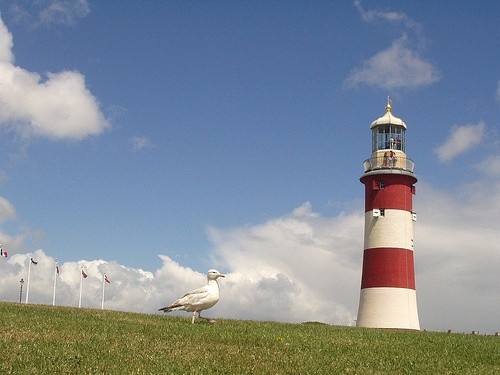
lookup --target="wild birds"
[158,269,225,323]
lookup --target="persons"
[385,137,402,150]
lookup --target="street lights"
[19,278,24,303]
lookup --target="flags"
[0,248,8,257]
[82,270,87,278]
[30,257,38,265]
[104,275,110,284]
[57,264,59,275]
